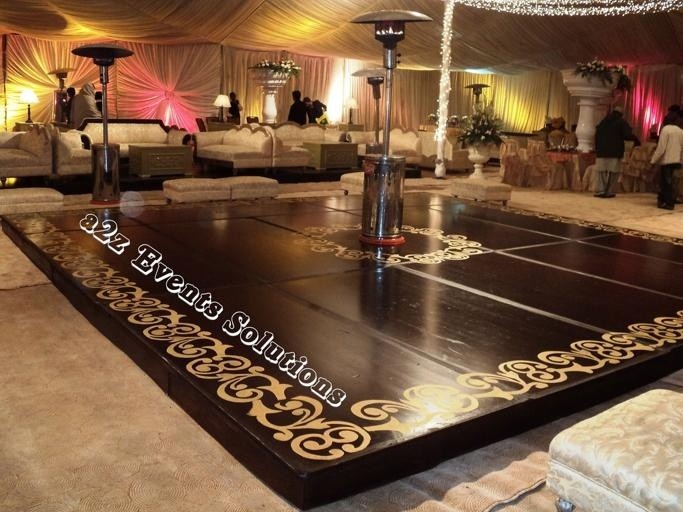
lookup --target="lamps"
[48,68,77,121]
[17,89,41,123]
[71,42,135,202]
[350,68,387,155]
[463,83,490,104]
[211,94,232,122]
[348,8,435,246]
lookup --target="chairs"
[499,130,664,192]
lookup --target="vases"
[256,80,289,124]
[467,144,491,178]
[559,67,621,153]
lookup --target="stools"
[340,170,364,195]
[216,175,280,202]
[0,187,65,216]
[545,387,683,512]
[162,177,231,204]
[450,178,512,206]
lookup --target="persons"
[648,111,683,210]
[593,106,641,198]
[288,89,308,123]
[63,85,76,122]
[650,104,683,144]
[303,98,329,128]
[67,83,101,130]
[540,115,579,149]
[93,92,103,112]
[226,91,244,123]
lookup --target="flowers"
[573,55,633,95]
[454,104,505,149]
[247,54,302,81]
